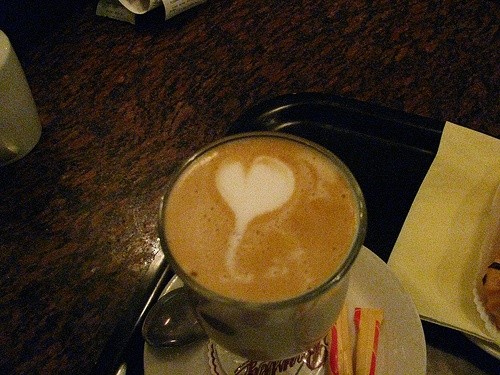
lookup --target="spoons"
[141,287,207,348]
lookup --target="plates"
[144,243,427,375]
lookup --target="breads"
[481,238,500,331]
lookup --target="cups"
[0,29,42,167]
[158,132,366,361]
